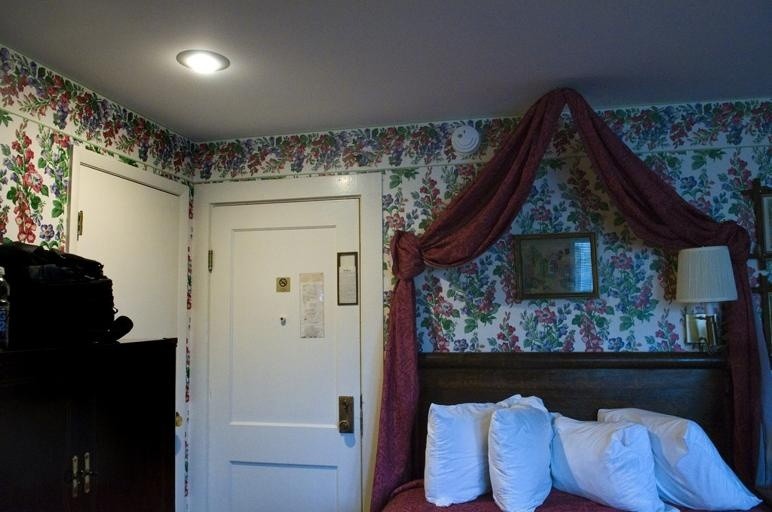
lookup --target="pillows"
[548,410,681,512]
[487,395,553,512]
[596,406,764,510]
[423,393,523,507]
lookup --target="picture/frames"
[512,231,600,299]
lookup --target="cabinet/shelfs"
[0,336,177,512]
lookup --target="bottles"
[0,265,12,352]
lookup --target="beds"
[378,349,772,512]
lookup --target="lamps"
[675,245,739,353]
